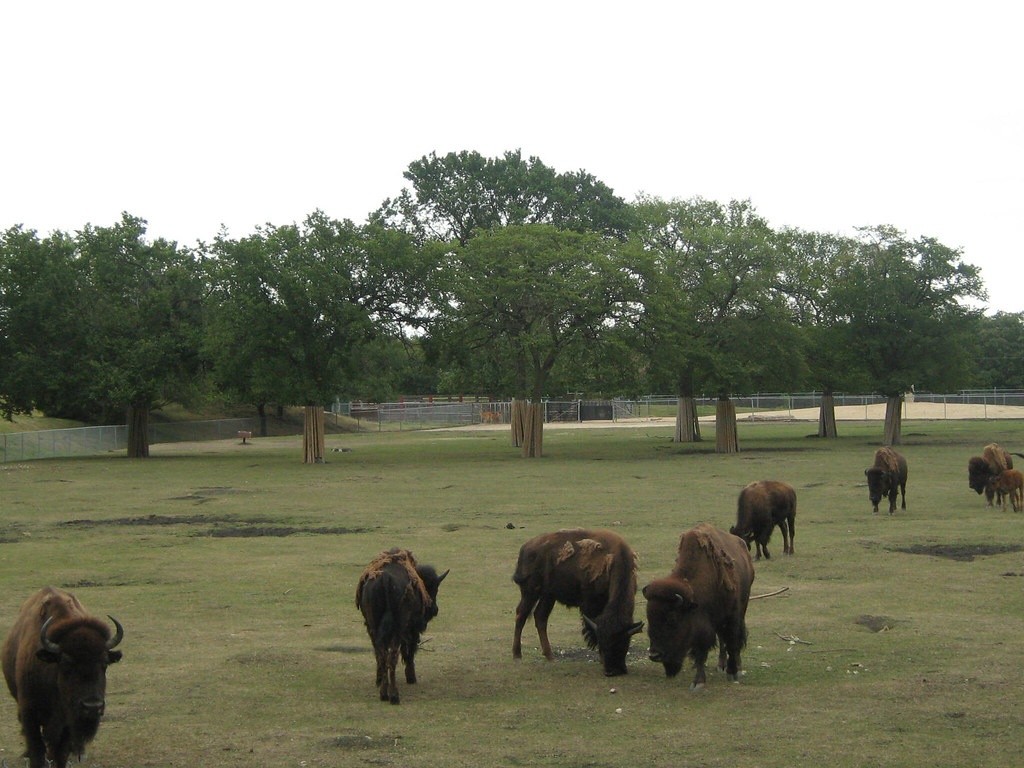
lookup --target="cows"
[968,441,1023,515]
[1,583,125,768]
[863,449,909,514]
[512,526,646,678]
[355,546,452,705]
[641,525,757,693]
[728,479,800,560]
